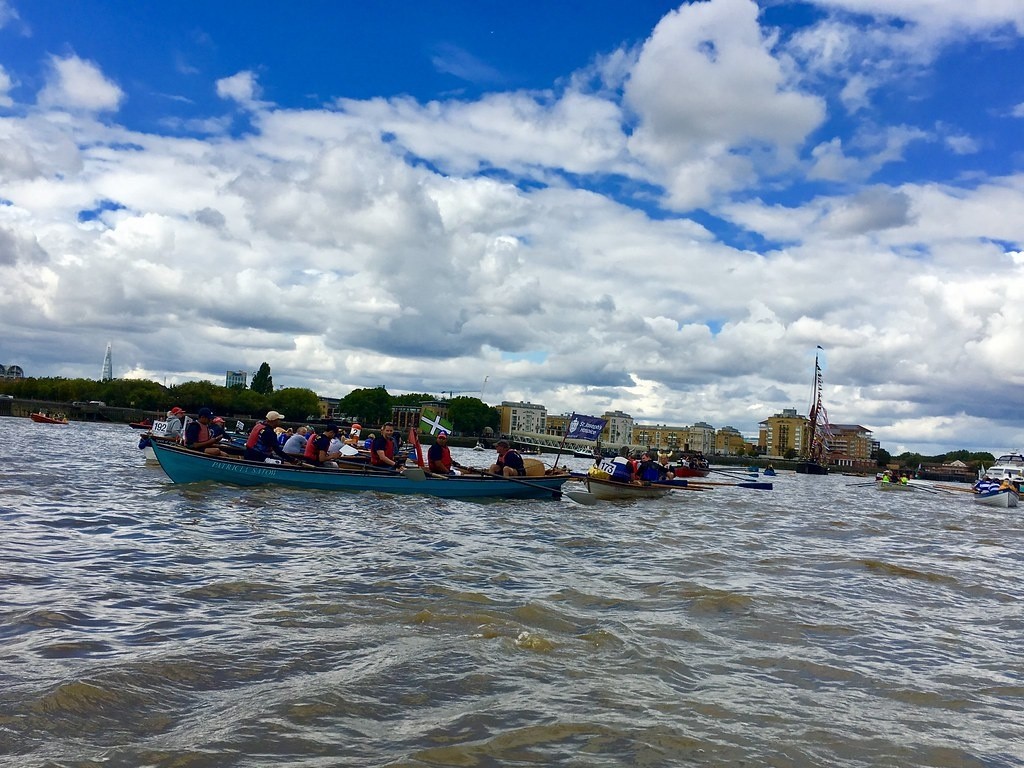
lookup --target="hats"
[368,434,375,438]
[438,430,450,435]
[657,450,673,458]
[680,455,687,459]
[172,407,185,415]
[307,427,315,431]
[212,417,226,423]
[265,410,286,421]
[198,407,216,418]
[493,440,509,448]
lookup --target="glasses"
[438,437,447,440]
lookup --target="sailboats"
[791,345,832,474]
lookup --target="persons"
[490,440,526,477]
[679,456,708,469]
[140,407,410,475]
[428,430,472,477]
[882,469,908,485]
[975,473,1017,496]
[592,446,676,483]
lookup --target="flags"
[566,411,608,441]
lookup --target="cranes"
[440,389,484,400]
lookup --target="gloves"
[292,458,304,465]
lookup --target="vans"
[73,402,87,408]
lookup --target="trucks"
[90,400,106,407]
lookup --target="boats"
[670,465,711,478]
[764,464,776,477]
[517,449,542,455]
[129,421,153,430]
[976,451,1024,495]
[138,433,157,460]
[973,488,1020,508]
[148,435,571,498]
[578,474,675,502]
[877,480,915,492]
[30,410,69,424]
[573,449,596,459]
[841,459,868,477]
[472,446,485,451]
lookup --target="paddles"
[451,462,596,506]
[846,482,877,490]
[907,482,951,495]
[932,484,977,494]
[650,464,775,494]
[333,456,428,483]
